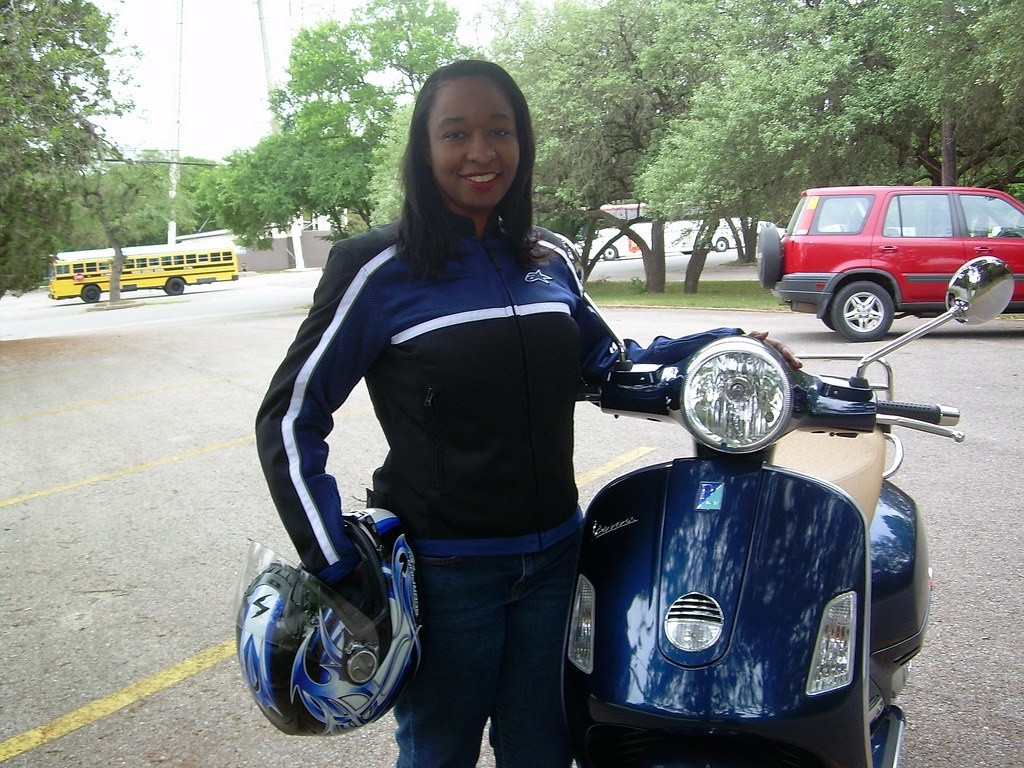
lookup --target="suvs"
[756,185,1024,342]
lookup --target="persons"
[255,59,802,768]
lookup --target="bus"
[48,235,240,304]
[574,202,756,262]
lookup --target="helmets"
[235,508,422,736]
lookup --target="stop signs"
[75,273,83,281]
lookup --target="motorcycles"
[545,230,1017,768]
